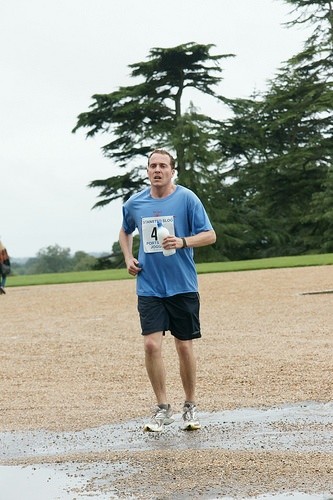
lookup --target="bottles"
[156,220,177,256]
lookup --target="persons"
[118,151,216,431]
[0,242,10,294]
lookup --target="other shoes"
[0,287,6,295]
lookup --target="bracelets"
[181,237,186,248]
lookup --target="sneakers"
[144,403,175,432]
[180,403,201,431]
